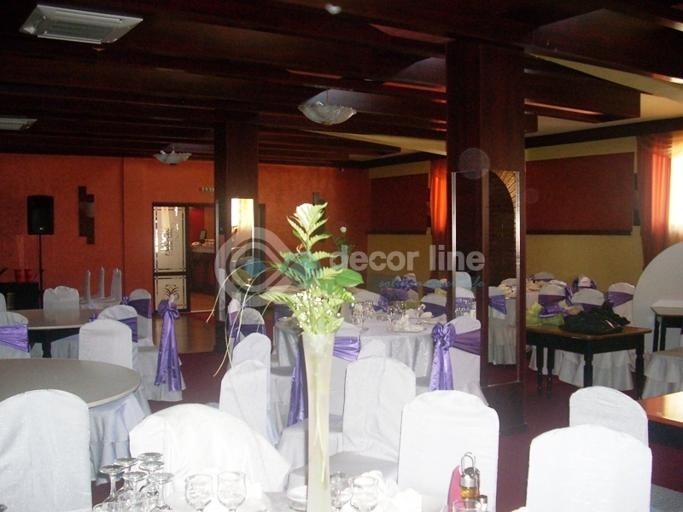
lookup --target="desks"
[274,312,443,379]
[649,306,682,352]
[525,322,651,401]
[9,309,101,358]
[0,358,152,486]
[642,391,682,427]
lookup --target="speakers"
[26,194,53,235]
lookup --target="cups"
[451,500,481,512]
[350,301,406,332]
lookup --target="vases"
[299,329,335,512]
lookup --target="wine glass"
[349,475,378,512]
[285,467,306,512]
[329,472,354,512]
[137,454,162,465]
[119,470,146,512]
[114,457,136,492]
[139,462,164,475]
[182,475,214,512]
[146,472,173,512]
[215,471,246,512]
[92,465,124,512]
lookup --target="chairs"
[95,304,137,368]
[78,318,132,369]
[525,270,634,322]
[126,288,153,347]
[227,299,241,359]
[0,292,6,312]
[0,311,30,359]
[567,386,681,512]
[219,359,267,438]
[286,354,416,512]
[524,425,654,512]
[237,308,293,413]
[427,315,482,399]
[30,286,80,356]
[276,317,360,470]
[397,389,499,512]
[128,403,290,494]
[135,294,186,403]
[488,278,516,365]
[231,332,271,374]
[0,389,93,512]
[641,349,682,400]
[340,270,477,318]
[81,266,122,309]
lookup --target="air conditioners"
[19,4,143,44]
[0,117,37,131]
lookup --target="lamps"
[297,85,357,125]
[152,144,191,166]
